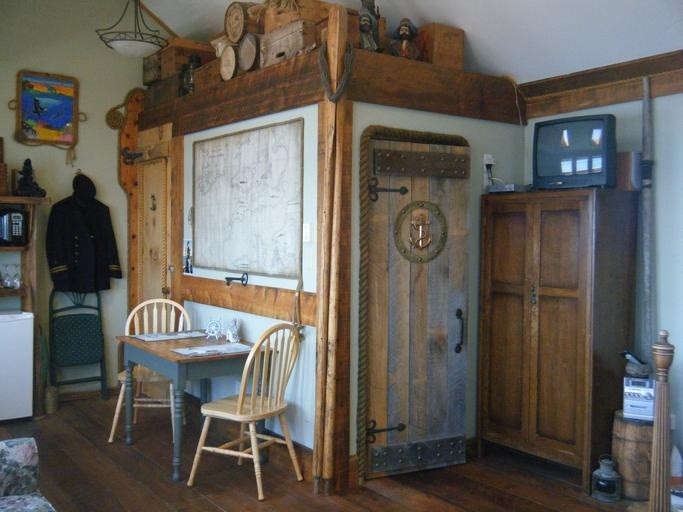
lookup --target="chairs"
[188,323,303,501]
[49,289,109,399]
[107,298,192,442]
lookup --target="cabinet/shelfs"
[477,186,640,496]
[0,194,52,424]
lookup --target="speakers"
[617,152,641,191]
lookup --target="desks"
[117,330,279,482]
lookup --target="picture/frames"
[14,70,79,148]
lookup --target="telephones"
[0,208,29,247]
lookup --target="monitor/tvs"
[532,114,617,189]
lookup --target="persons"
[357,13,378,52]
[390,17,420,58]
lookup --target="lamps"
[96,1,171,59]
